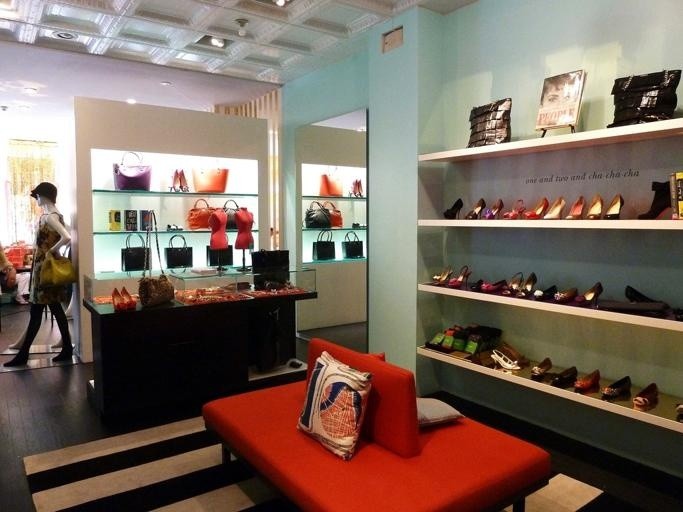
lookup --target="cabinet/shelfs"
[89,143,262,280]
[416,116,682,436]
[301,162,366,265]
[87,267,318,428]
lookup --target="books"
[108,208,121,231]
[139,210,152,231]
[123,210,137,231]
[669,171,683,220]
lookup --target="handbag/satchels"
[40,252,76,288]
[605,70,682,126]
[319,166,343,196]
[467,98,512,148]
[306,200,363,260]
[109,151,288,310]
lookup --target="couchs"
[202,338,551,512]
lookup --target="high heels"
[443,182,672,218]
[425,265,683,322]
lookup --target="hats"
[31,182,57,204]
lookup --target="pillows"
[416,395,464,427]
[298,351,371,462]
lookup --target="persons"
[8,223,75,350]
[0,243,30,306]
[536,72,581,125]
[235,207,254,249]
[208,208,228,250]
[4,182,74,367]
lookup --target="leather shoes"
[491,340,683,415]
[358,180,366,199]
[351,179,361,199]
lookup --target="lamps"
[235,18,250,37]
[274,1,289,7]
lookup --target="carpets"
[21,415,604,512]
